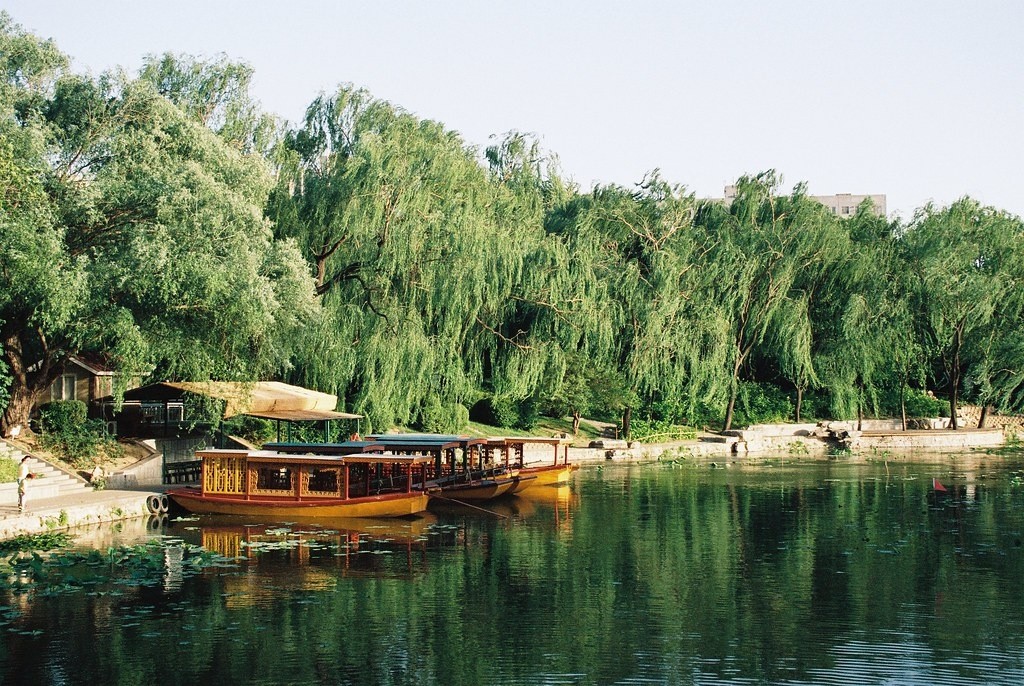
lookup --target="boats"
[163,433,572,518]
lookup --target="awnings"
[122,382,361,444]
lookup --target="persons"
[349,432,362,442]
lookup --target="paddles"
[425,492,509,519]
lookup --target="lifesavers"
[159,494,170,512]
[147,495,161,513]
[159,513,169,529]
[146,514,161,532]
